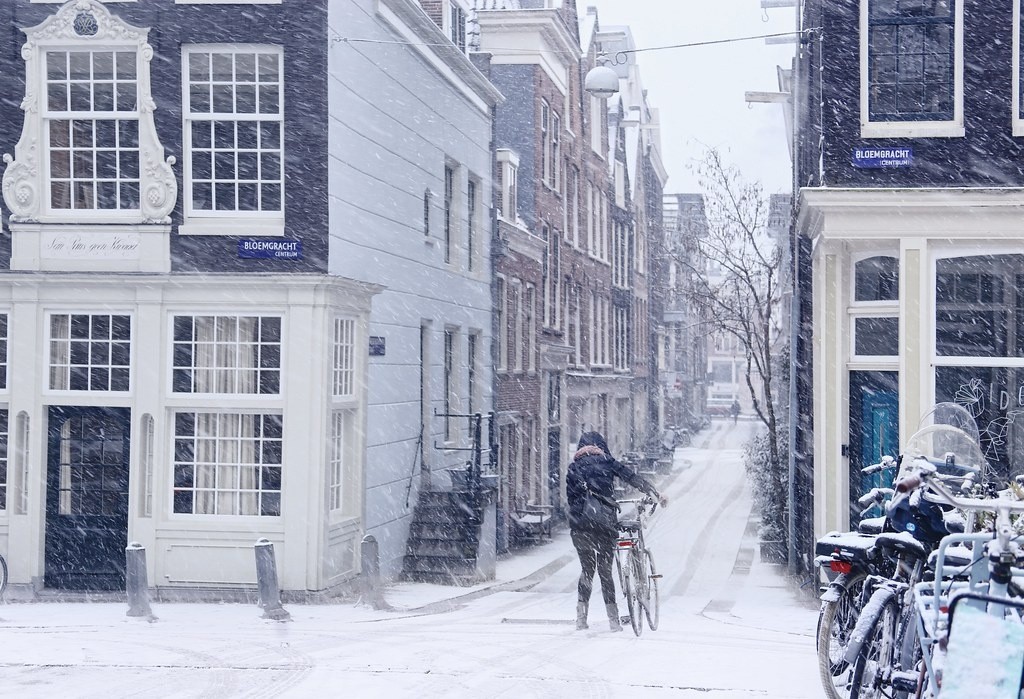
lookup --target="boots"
[606,603,623,633]
[576,602,590,630]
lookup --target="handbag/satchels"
[579,489,621,538]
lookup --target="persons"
[731,399,740,425]
[567,431,668,632]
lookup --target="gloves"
[648,487,669,507]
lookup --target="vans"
[707,399,738,418]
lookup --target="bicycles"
[666,423,691,448]
[812,399,1024,699]
[613,495,664,637]
